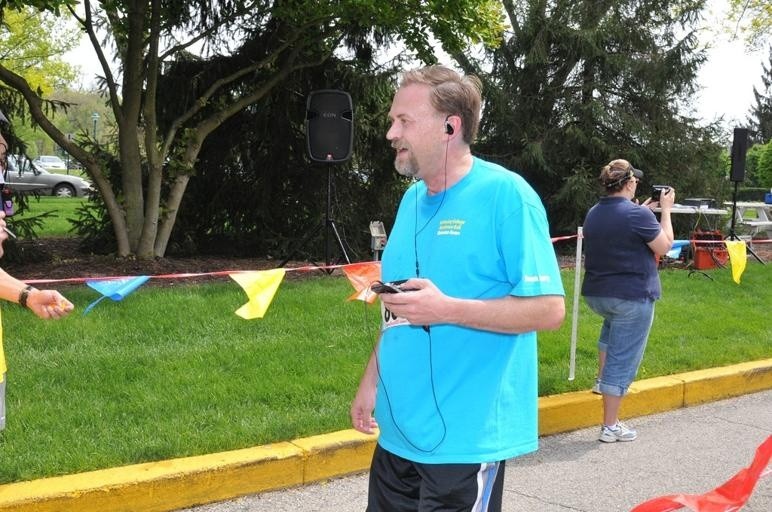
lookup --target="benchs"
[741,222,772,241]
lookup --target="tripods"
[276,164,352,276]
[723,181,765,265]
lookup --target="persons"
[582,158,676,444]
[346,66,568,512]
[0,135,76,439]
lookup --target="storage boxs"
[764,193,772,204]
[691,230,728,270]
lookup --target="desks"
[725,201,772,228]
[654,208,728,235]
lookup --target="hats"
[600,162,644,187]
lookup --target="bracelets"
[19,285,34,310]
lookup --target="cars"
[32,155,68,171]
[2,155,99,198]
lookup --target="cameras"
[652,185,669,201]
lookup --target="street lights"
[91,113,101,139]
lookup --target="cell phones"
[371,283,403,294]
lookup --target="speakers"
[307,90,354,164]
[730,128,747,181]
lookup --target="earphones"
[446,123,454,135]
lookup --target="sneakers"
[599,420,638,443]
[592,380,602,395]
